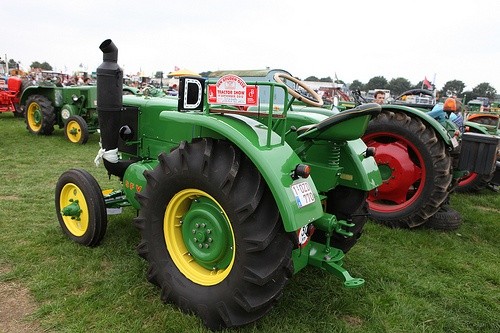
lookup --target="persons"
[9,69,180,97]
[374,91,385,104]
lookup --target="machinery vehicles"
[0,38,500,332]
